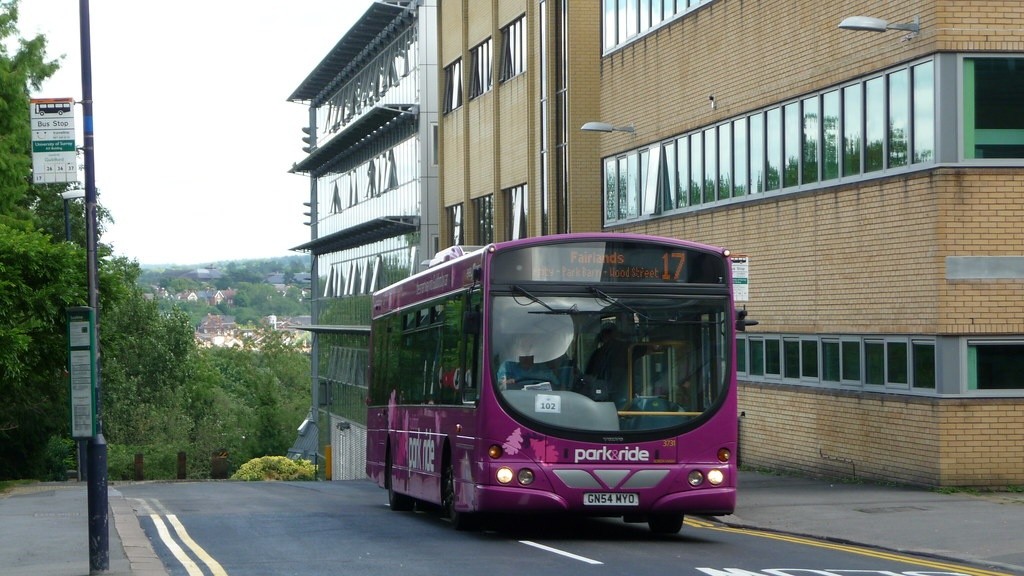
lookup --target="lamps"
[581,121,635,133]
[838,16,919,36]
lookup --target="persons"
[584,322,649,386]
[543,329,574,391]
[497,334,560,389]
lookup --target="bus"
[366,231,759,538]
